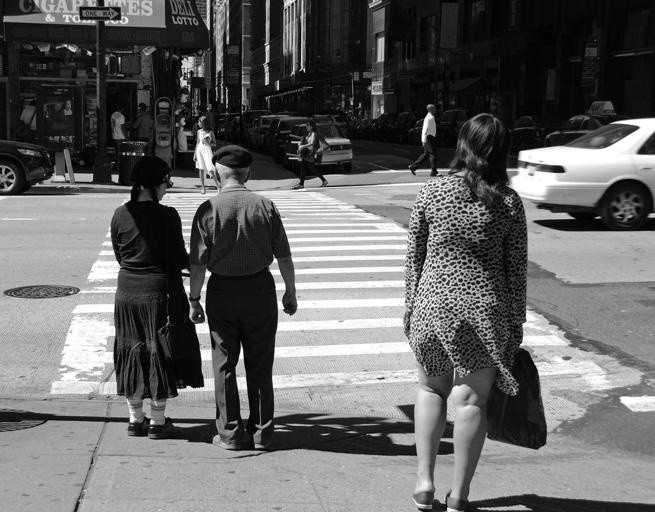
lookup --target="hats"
[212,144,254,169]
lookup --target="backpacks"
[312,134,323,163]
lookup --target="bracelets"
[190,295,201,302]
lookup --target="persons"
[111,106,126,164]
[188,144,297,451]
[20,100,37,143]
[404,114,528,512]
[409,103,443,176]
[111,156,204,440]
[64,100,74,116]
[132,103,153,142]
[193,116,220,194]
[178,112,187,152]
[293,121,329,188]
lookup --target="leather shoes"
[408,165,416,176]
[430,172,442,177]
[291,183,304,190]
[321,180,329,187]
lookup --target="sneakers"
[128,416,150,437]
[148,418,181,438]
[413,490,435,511]
[252,431,274,450]
[210,430,242,450]
[445,490,469,512]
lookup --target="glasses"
[163,177,174,188]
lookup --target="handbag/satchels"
[484,348,547,451]
[297,133,312,158]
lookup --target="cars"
[367,107,548,149]
[508,116,655,230]
[0,138,55,196]
[216,110,358,158]
[287,122,354,173]
[543,98,634,148]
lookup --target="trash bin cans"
[118,140,148,186]
